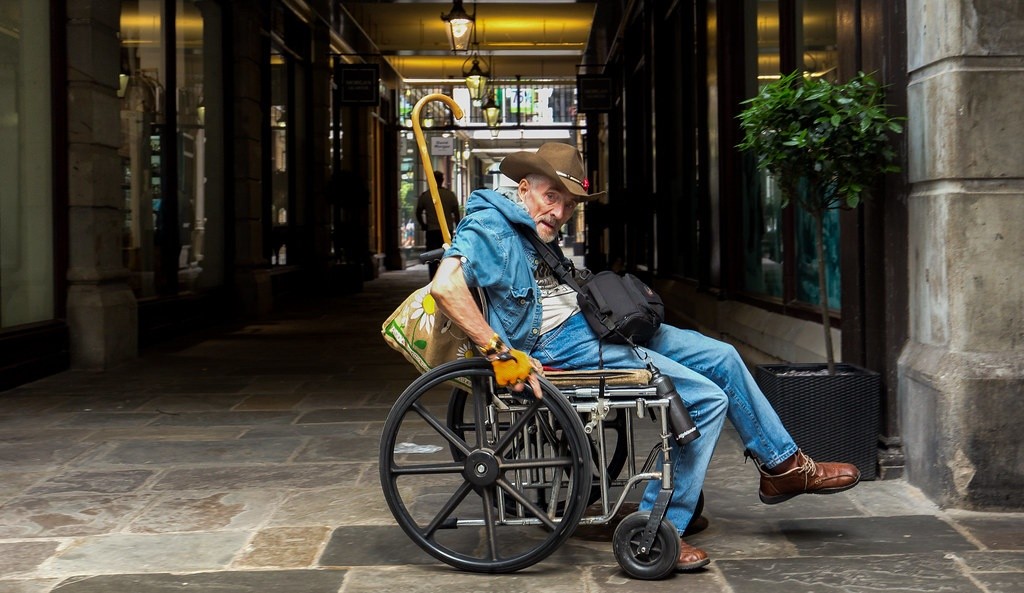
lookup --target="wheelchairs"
[376,247,682,581]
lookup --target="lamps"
[439,0,476,52]
[490,117,503,137]
[482,65,501,127]
[462,28,492,107]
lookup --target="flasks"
[647,362,701,447]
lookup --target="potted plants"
[733,66,909,482]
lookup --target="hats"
[498,141,607,203]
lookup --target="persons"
[430,141,861,570]
[416,170,461,282]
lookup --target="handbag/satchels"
[380,275,475,398]
[576,271,666,347]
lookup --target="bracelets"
[479,332,519,365]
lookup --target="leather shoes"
[676,540,711,570]
[758,447,862,506]
